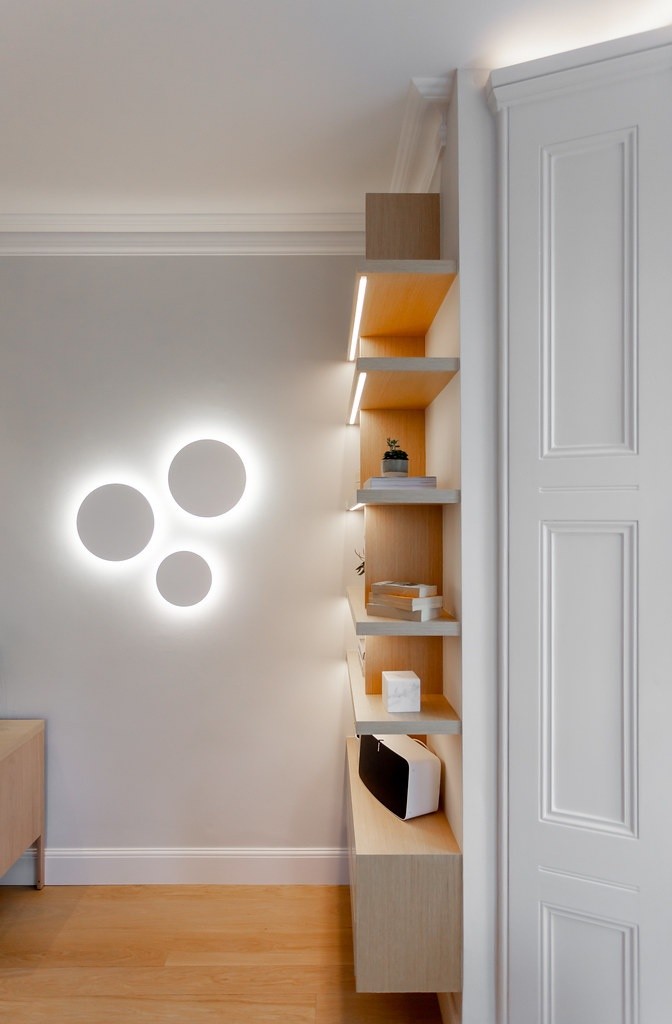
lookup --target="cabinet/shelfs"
[347,258,464,995]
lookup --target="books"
[367,581,443,622]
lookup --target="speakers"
[359,733,441,820]
